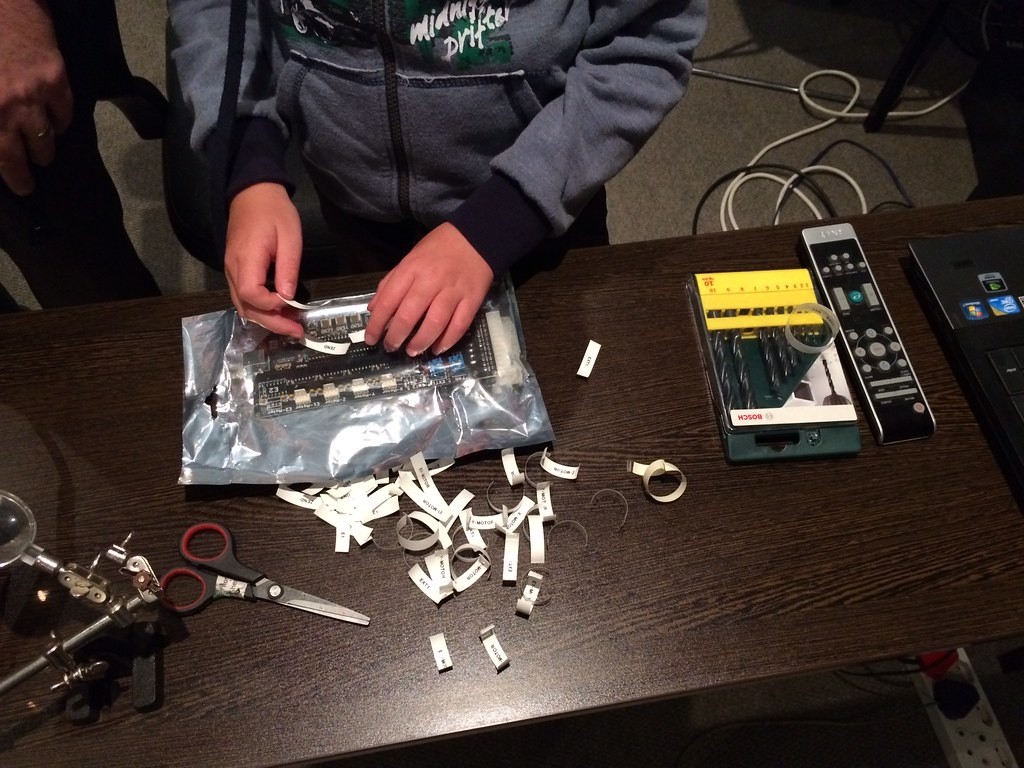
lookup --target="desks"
[0,194,1024,768]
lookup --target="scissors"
[157,521,372,629]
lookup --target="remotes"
[802,222,936,445]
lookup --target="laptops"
[907,230,1024,462]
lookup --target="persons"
[0,0,161,309]
[163,0,709,355]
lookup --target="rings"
[33,124,49,142]
